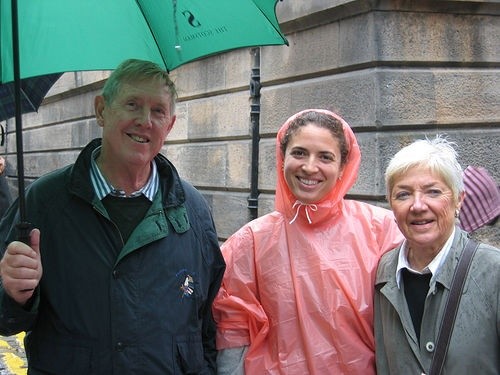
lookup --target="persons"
[0,58,227,375]
[212,107,408,375]
[373,136,500,375]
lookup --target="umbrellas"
[0,0,290,243]
[0,70,65,122]
